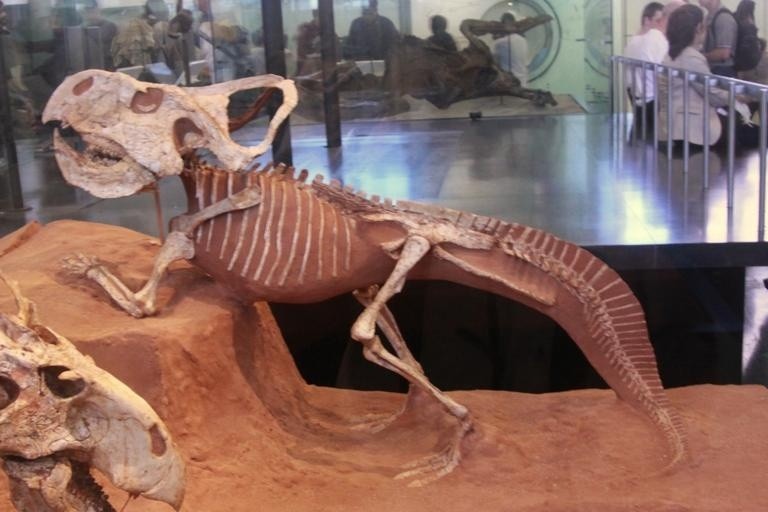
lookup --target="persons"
[426,13,456,53]
[621,1,768,157]
[342,1,401,63]
[488,14,529,89]
[295,7,322,77]
[2,1,290,126]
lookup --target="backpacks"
[731,10,763,72]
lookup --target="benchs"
[680,148,722,175]
